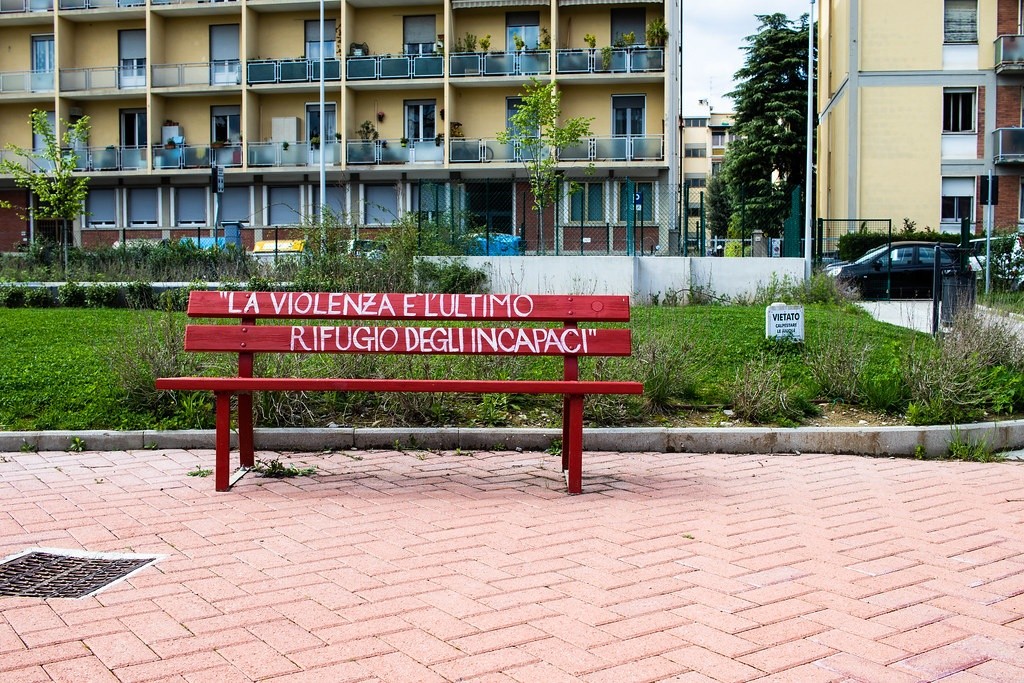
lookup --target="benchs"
[251,237,314,270]
[156,291,644,496]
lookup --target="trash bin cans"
[942,269,976,323]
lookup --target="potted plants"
[279,133,342,154]
[454,28,552,76]
[164,139,176,150]
[358,119,445,162]
[211,140,225,148]
[558,17,669,72]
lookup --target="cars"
[823,242,977,300]
[956,236,1024,281]
